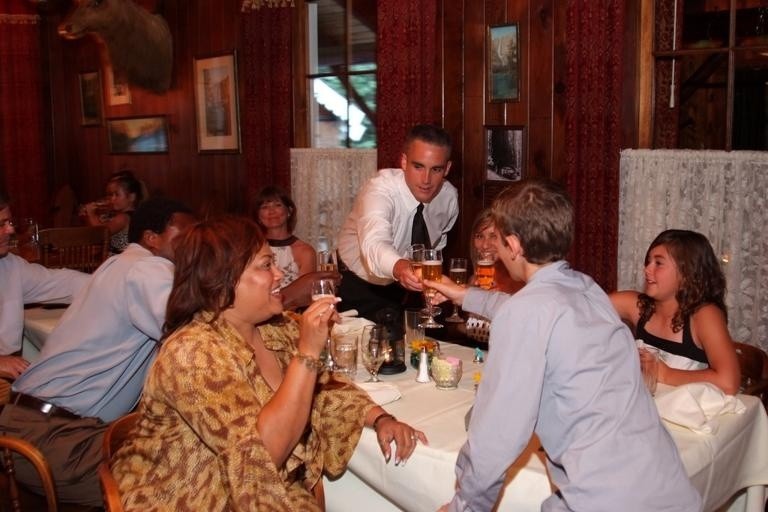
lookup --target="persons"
[112,212,430,512]
[0,187,93,408]
[605,227,743,397]
[326,122,460,346]
[0,193,345,512]
[77,170,149,260]
[419,175,704,512]
[253,182,317,289]
[459,209,526,350]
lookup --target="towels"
[653,380,747,436]
[331,308,377,337]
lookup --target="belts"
[8,391,81,427]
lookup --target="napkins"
[354,379,402,407]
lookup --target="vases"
[416,350,431,383]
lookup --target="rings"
[411,435,420,441]
[378,438,390,445]
[318,313,324,318]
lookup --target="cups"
[10,218,41,256]
[638,347,662,398]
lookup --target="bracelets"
[294,351,323,372]
[373,413,397,432]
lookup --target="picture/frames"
[105,113,170,155]
[191,49,242,155]
[104,61,132,105]
[482,124,525,187]
[484,20,520,103]
[78,66,106,128]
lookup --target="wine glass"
[79,199,114,217]
[312,242,498,393]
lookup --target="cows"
[54,0,177,96]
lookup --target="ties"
[410,201,433,262]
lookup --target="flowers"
[409,337,436,352]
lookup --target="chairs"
[97,411,137,512]
[38,224,111,273]
[0,434,56,512]
[734,341,767,414]
